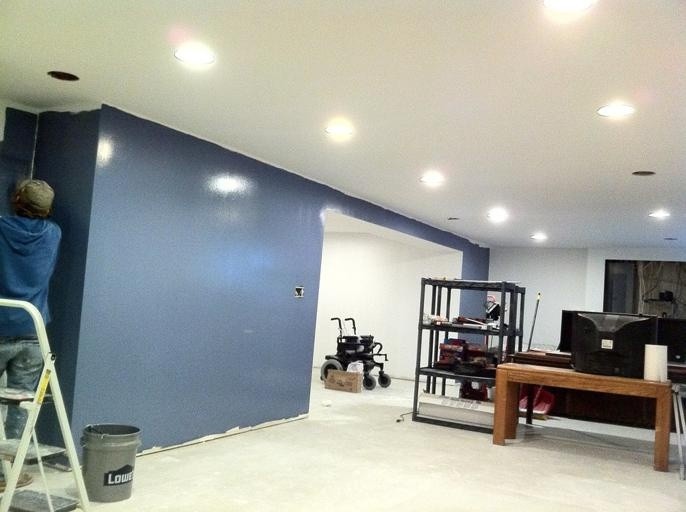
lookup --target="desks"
[492,350,686,473]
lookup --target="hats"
[13,175,56,212]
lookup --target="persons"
[0,179,62,491]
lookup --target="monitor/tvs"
[575,313,659,379]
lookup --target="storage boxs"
[325,368,364,393]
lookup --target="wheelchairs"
[320,317,390,390]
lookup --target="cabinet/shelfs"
[411,278,526,433]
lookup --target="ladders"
[0,297,91,512]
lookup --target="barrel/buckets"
[79,423,141,503]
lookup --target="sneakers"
[0,472,34,493]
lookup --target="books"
[429,314,498,371]
